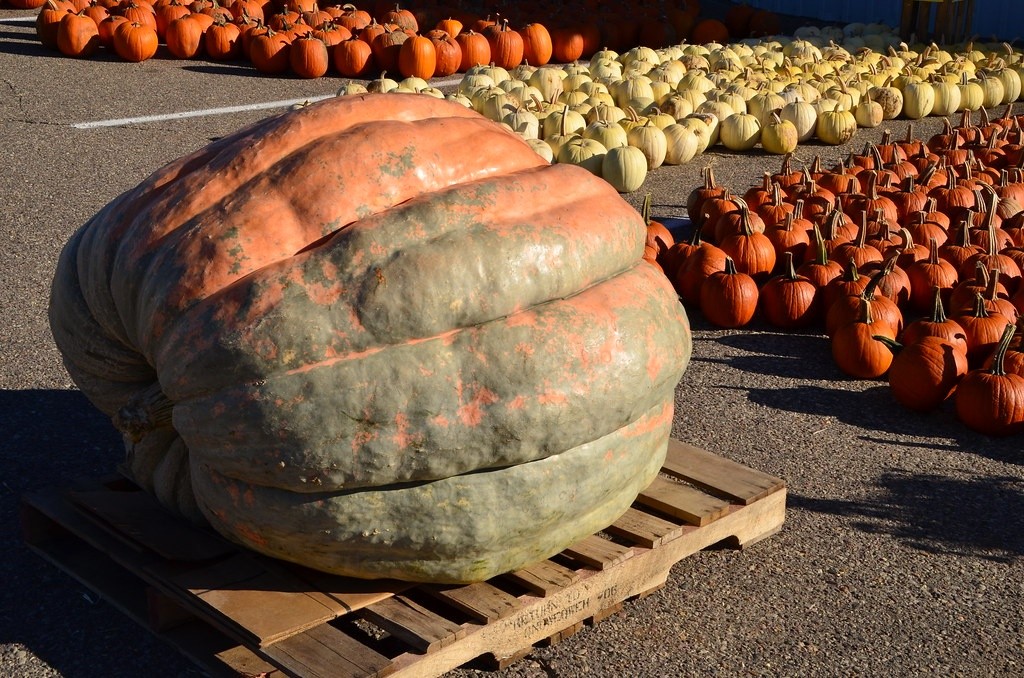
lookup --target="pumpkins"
[636,104,1024,442]
[8,0,1024,195]
[47,94,692,584]
